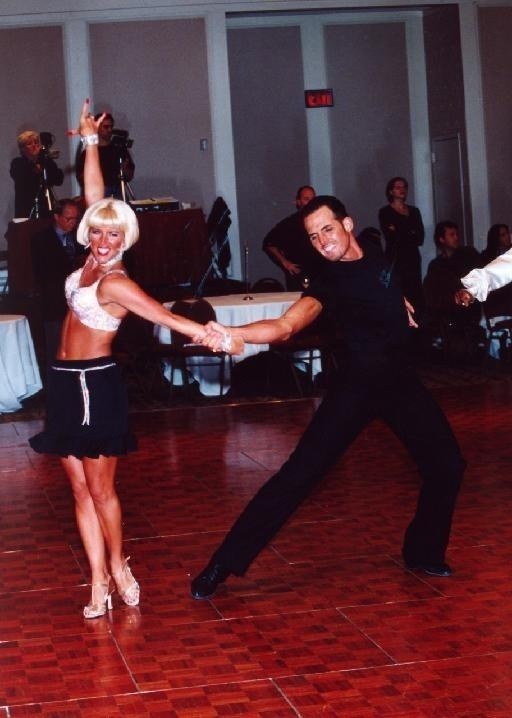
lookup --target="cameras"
[37,132,60,159]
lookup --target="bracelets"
[219,333,232,354]
[80,133,98,146]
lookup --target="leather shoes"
[407,561,453,576]
[191,550,231,600]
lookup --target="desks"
[0,309,45,414]
[8,202,215,298]
[151,291,321,399]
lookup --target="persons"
[480,223,512,370]
[9,130,64,220]
[25,198,90,394]
[28,97,244,620]
[421,219,482,362]
[452,246,511,309]
[262,186,323,292]
[379,177,424,350]
[190,194,464,598]
[75,112,135,208]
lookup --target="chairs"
[166,298,243,406]
[198,193,233,289]
[248,277,284,293]
[478,290,511,373]
[261,327,348,398]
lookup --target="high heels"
[112,555,140,605]
[84,575,117,618]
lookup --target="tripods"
[110,163,135,202]
[28,171,56,222]
